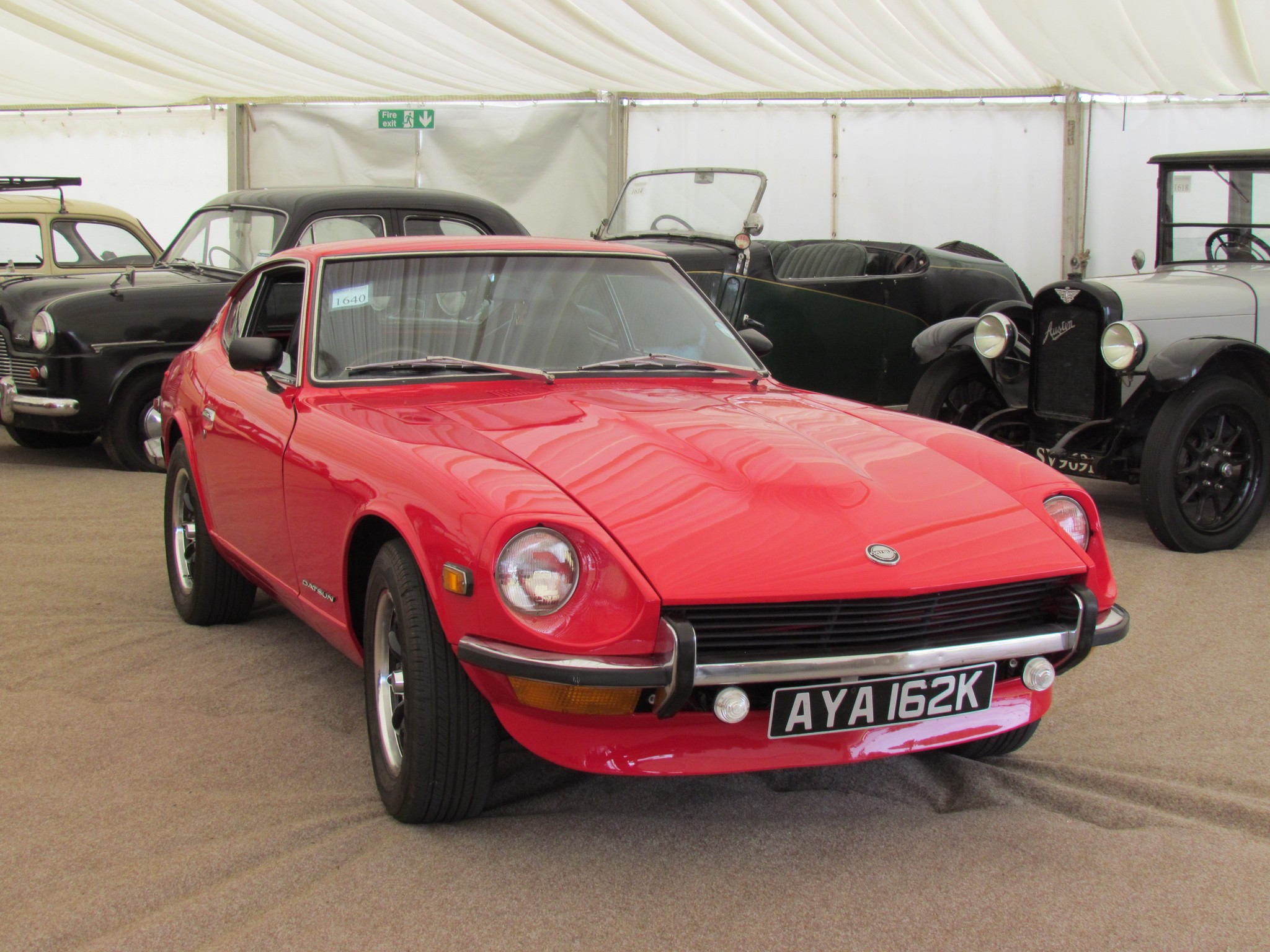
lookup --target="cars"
[1,193,168,283]
[368,166,1034,411]
[1,191,533,470]
[145,234,1129,826]
[905,152,1269,553]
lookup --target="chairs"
[521,296,597,371]
[777,242,869,280]
[313,293,379,383]
[750,239,797,275]
